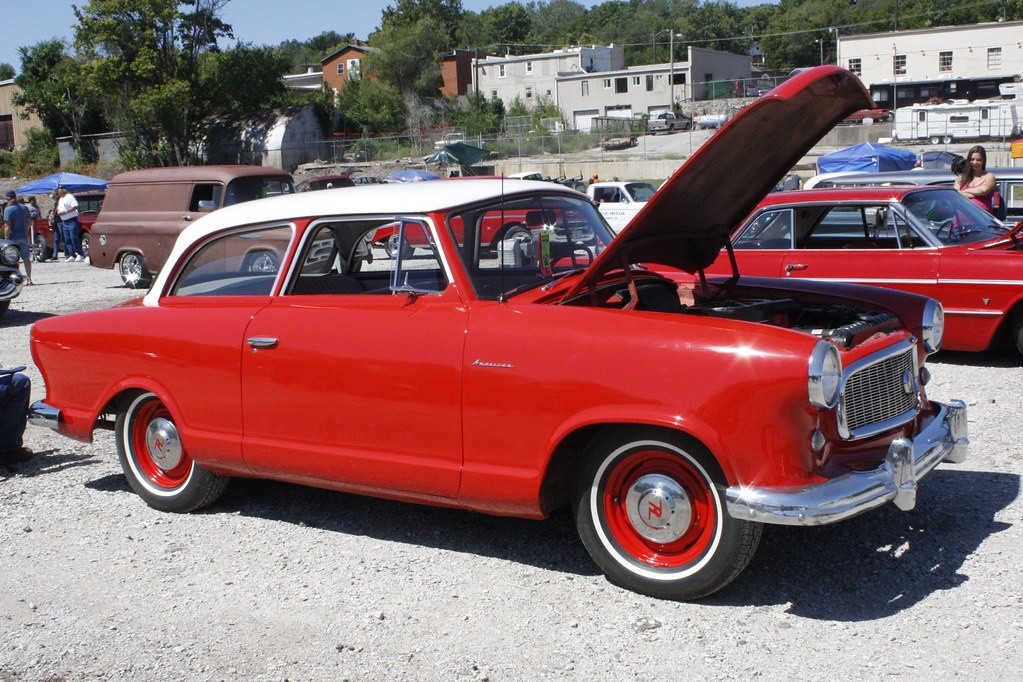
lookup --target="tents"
[18,172,110,195]
[426,142,490,179]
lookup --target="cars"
[293,171,440,194]
[694,186,1023,366]
[913,151,968,172]
[0,239,26,310]
[368,176,581,260]
[29,66,969,601]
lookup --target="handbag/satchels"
[48,210,55,231]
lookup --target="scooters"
[546,172,586,193]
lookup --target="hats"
[6,190,15,197]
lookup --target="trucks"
[586,181,655,234]
[870,74,1023,145]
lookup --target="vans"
[87,167,374,289]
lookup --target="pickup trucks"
[31,191,106,263]
[645,113,696,135]
[843,109,891,123]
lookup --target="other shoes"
[2,447,33,466]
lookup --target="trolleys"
[600,136,638,149]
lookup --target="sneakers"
[65,256,75,262]
[74,255,84,262]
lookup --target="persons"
[17,195,42,219]
[950,145,996,232]
[0,363,34,462]
[2,190,32,285]
[589,174,620,183]
[49,188,84,263]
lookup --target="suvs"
[434,133,466,151]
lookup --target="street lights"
[829,28,841,66]
[815,38,826,66]
[652,30,668,63]
[669,28,683,114]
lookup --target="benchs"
[209,272,366,295]
[737,236,791,249]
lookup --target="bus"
[757,166,1022,241]
